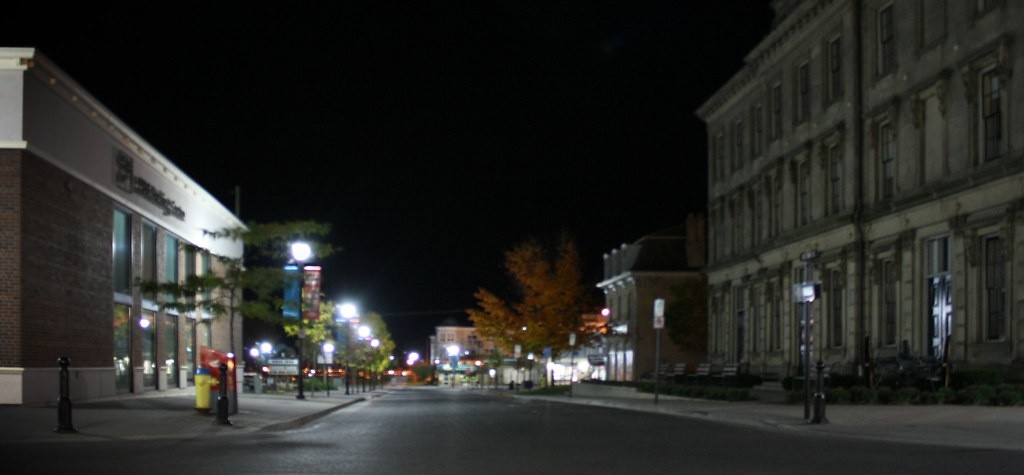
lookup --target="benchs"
[649,361,741,386]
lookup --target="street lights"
[370,339,379,390]
[259,341,274,393]
[338,301,357,395]
[322,342,335,399]
[357,325,371,390]
[449,343,459,389]
[291,241,310,400]
